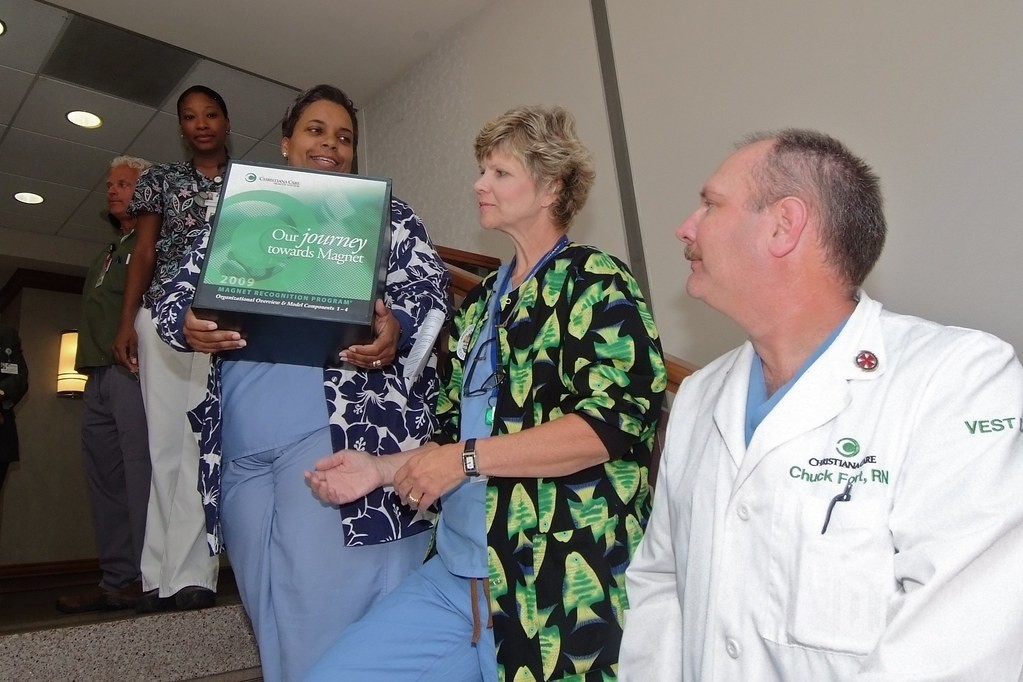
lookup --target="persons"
[617,127,1023,682]
[58,156,153,615]
[303,106,669,681]
[114,84,233,612]
[0,326,28,488]
[155,85,451,682]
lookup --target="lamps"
[58,330,90,398]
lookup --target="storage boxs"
[190,160,393,371]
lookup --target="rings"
[373,359,381,368]
[408,494,420,505]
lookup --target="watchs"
[462,438,481,478]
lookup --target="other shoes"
[175,584,216,611]
[53,582,141,612]
[110,588,178,613]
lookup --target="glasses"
[463,310,506,398]
